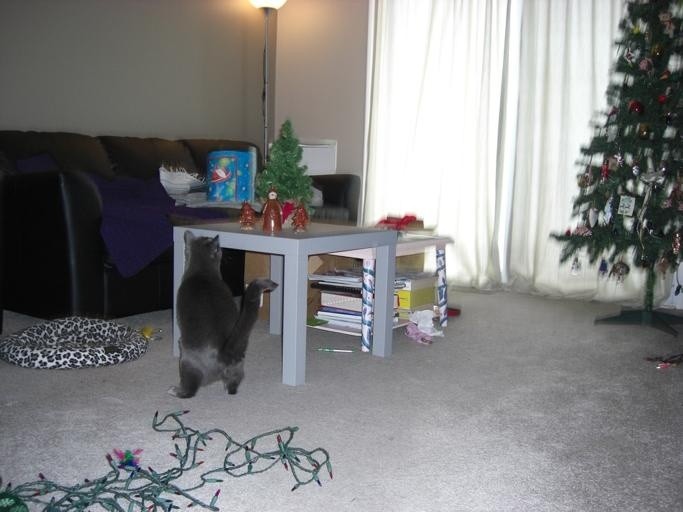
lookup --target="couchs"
[0,131,361,321]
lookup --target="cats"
[166,230,279,399]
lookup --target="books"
[309,270,400,330]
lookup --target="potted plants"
[253,119,314,228]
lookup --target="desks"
[171,216,399,386]
[328,236,455,352]
